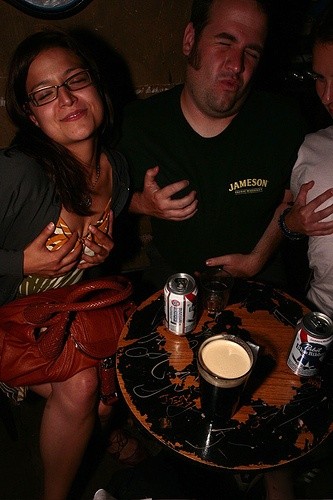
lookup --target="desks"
[115,277,333,500]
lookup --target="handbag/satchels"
[0,275,136,388]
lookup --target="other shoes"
[234,471,264,496]
[102,431,148,466]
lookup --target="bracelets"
[278,208,305,241]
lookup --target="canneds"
[286,312,333,377]
[163,272,198,335]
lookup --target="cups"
[197,266,233,314]
[197,334,255,425]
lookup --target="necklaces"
[80,157,100,208]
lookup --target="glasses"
[247,281,300,318]
[24,68,93,107]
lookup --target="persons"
[0,28,134,500]
[116,0,291,307]
[276,21,333,318]
